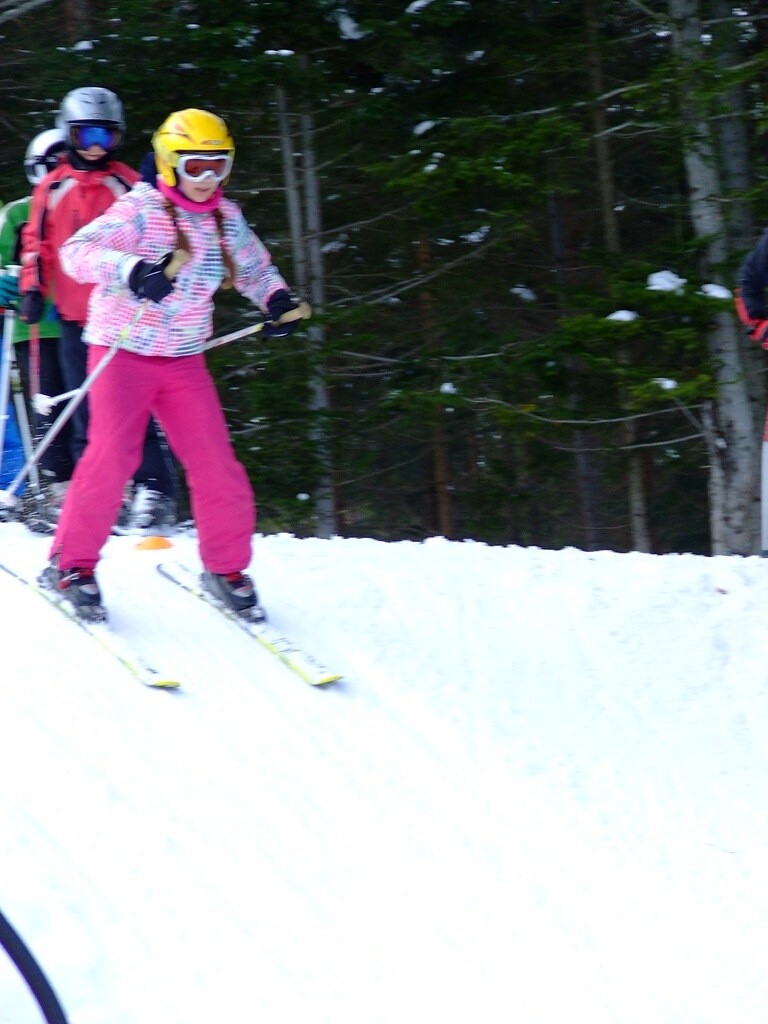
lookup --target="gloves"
[134,253,178,304]
[260,292,301,339]
[22,291,43,324]
[0,275,20,305]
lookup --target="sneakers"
[47,554,100,609]
[208,568,256,608]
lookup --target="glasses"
[176,154,234,182]
[54,152,66,168]
[69,123,124,152]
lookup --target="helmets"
[24,130,71,184]
[152,108,235,187]
[62,87,127,148]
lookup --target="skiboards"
[0,533,347,693]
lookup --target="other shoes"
[126,488,177,529]
[0,509,52,533]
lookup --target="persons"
[47,107,302,613]
[1,88,175,539]
[733,227,768,349]
